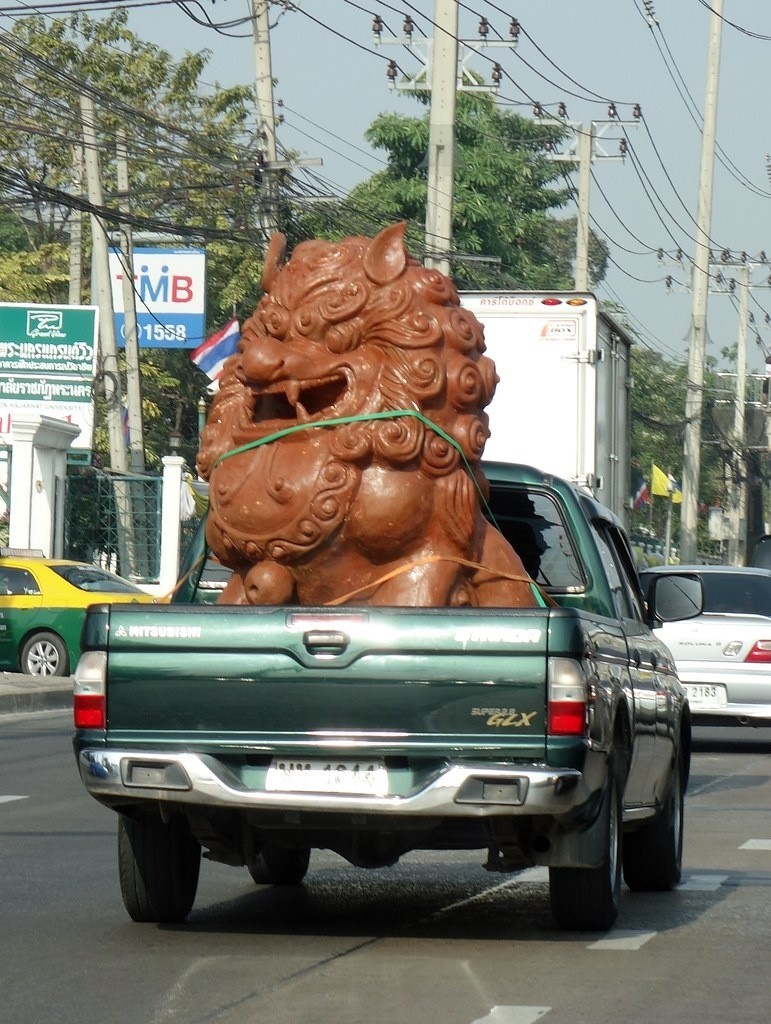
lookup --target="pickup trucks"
[71,460,706,931]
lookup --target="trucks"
[449,289,636,527]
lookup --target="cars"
[0,548,171,677]
[637,565,771,728]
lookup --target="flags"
[650,463,675,498]
[188,315,240,396]
[632,475,650,512]
[667,472,682,504]
[698,498,707,512]
